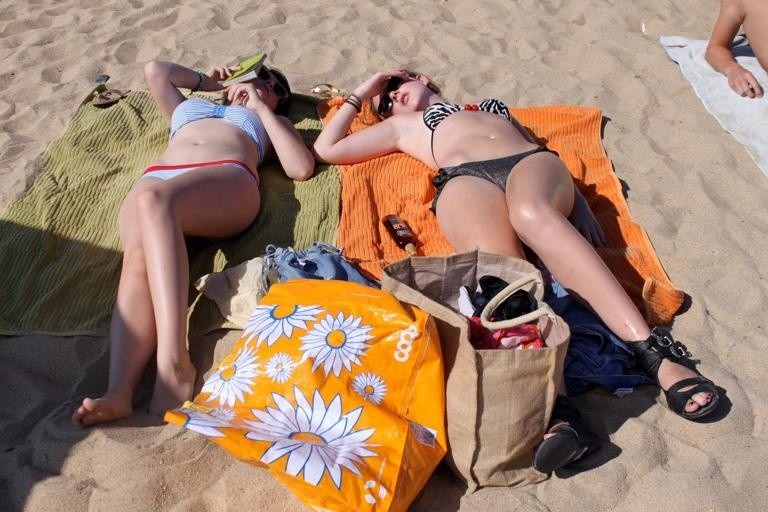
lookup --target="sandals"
[536,395,591,472]
[629,323,721,420]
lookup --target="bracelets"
[190,68,206,94]
[343,92,362,113]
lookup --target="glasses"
[377,76,404,119]
[258,68,289,98]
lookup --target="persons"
[705,1,767,98]
[68,60,318,427]
[313,68,725,473]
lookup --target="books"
[222,52,268,87]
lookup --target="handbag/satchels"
[165,279,446,512]
[261,244,380,289]
[192,256,268,336]
[382,251,570,493]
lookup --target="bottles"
[381,215,419,256]
